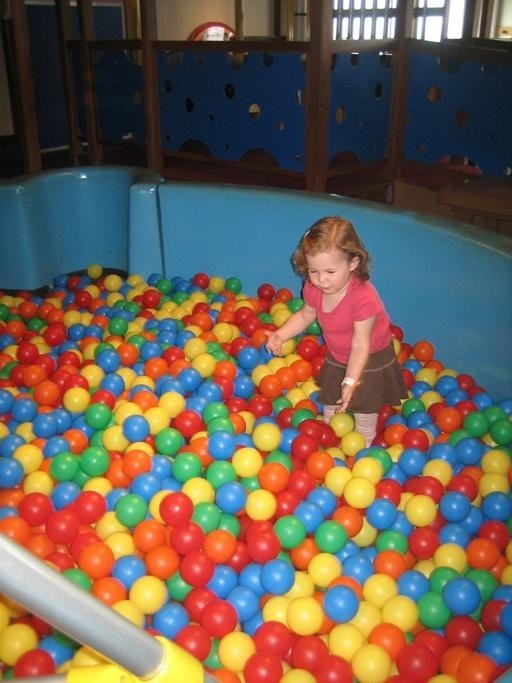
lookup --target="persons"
[265,214,409,448]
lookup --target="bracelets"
[339,374,363,393]
